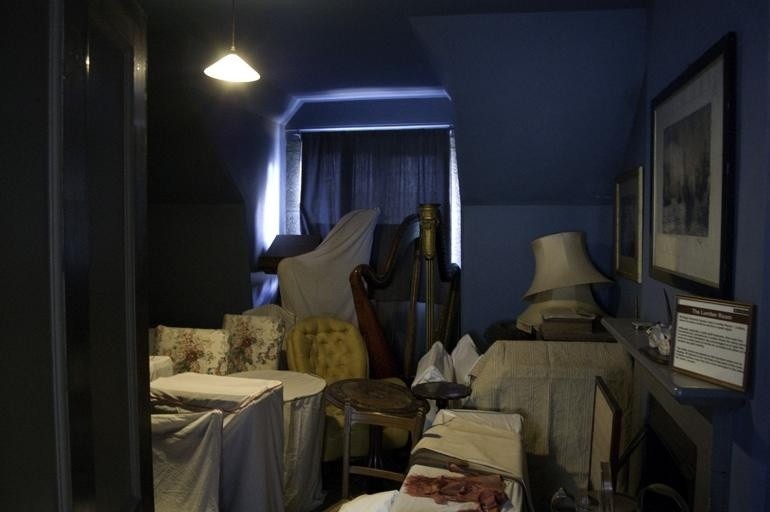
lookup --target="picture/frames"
[648,29,736,299]
[613,165,643,283]
[669,293,756,393]
[588,377,624,506]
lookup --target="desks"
[465,340,634,487]
[602,318,756,512]
[150,372,283,512]
[391,411,526,512]
[224,370,325,512]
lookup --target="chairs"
[325,398,427,511]
[223,314,282,372]
[286,315,374,460]
[154,325,232,374]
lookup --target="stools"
[413,383,472,409]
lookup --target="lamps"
[203,2,261,91]
